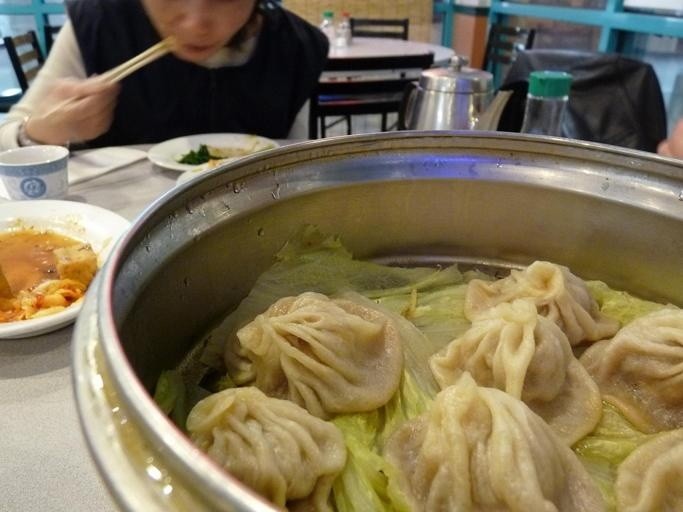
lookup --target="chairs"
[347,16,409,82]
[306,77,415,142]
[479,24,536,92]
[43,24,61,56]
[2,30,44,95]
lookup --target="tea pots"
[405,54,510,133]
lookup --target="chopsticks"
[90,39,180,91]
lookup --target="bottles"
[519,68,571,135]
[320,8,356,52]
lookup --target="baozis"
[385,370,615,511]
[429,298,603,448]
[615,428,680,511]
[578,309,682,435]
[464,261,623,347]
[185,386,346,511]
[223,292,403,421]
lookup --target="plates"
[0,200,135,339]
[149,132,281,174]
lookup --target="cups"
[0,143,70,199]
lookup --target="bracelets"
[18,115,44,146]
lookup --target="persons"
[656,118,683,160]
[0,0,329,150]
